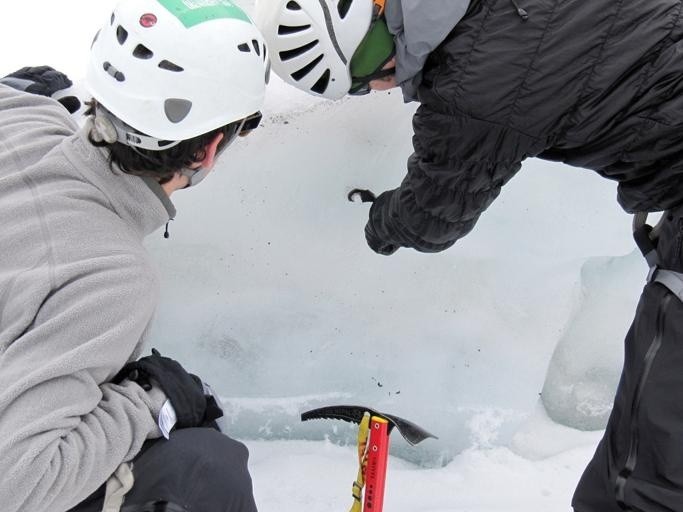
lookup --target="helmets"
[253,0,376,102]
[78,0,271,152]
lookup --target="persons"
[258,0,682,512]
[1,1,268,512]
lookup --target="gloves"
[0,65,82,115]
[364,190,407,257]
[133,347,224,434]
